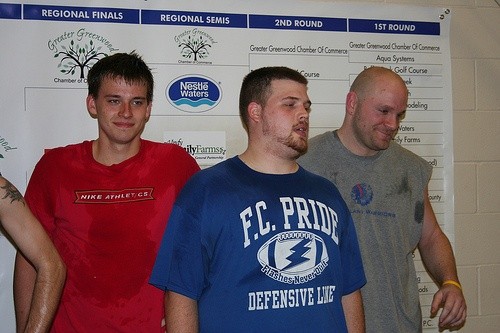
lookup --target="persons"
[0,172,68,333]
[148,66,368,333]
[13,49,203,333]
[295,65,467,333]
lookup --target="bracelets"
[440,280,462,291]
